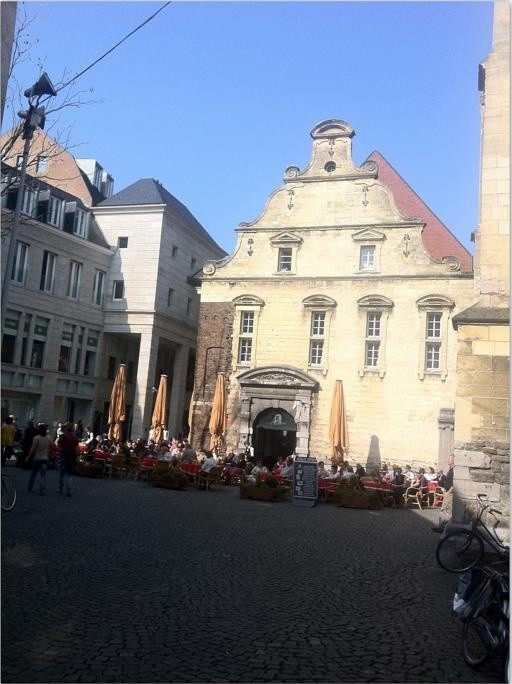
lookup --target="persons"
[441,454,454,511]
[57,422,64,437]
[272,456,294,480]
[251,461,267,475]
[244,440,255,458]
[96,434,246,490]
[25,425,52,497]
[56,423,78,498]
[85,425,96,449]
[76,419,84,439]
[374,462,446,509]
[2,417,15,463]
[318,461,365,490]
[24,421,39,455]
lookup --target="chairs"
[85,450,447,510]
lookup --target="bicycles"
[452,567,511,682]
[1,468,17,512]
[436,493,510,572]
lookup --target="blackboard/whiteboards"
[292,462,318,500]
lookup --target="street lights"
[2,71,57,326]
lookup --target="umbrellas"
[152,374,169,445]
[108,363,127,440]
[329,376,349,464]
[209,368,226,458]
